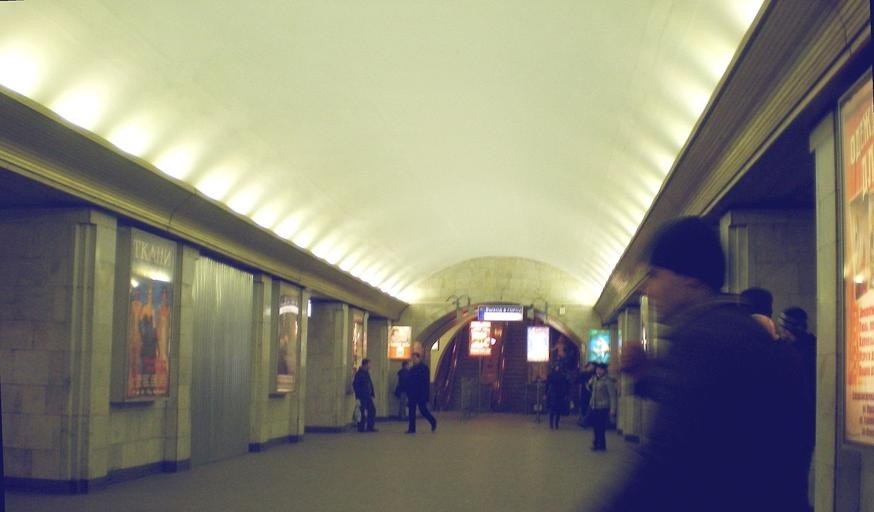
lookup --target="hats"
[650,215,724,289]
[740,289,772,318]
[778,307,808,335]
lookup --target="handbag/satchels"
[577,411,592,427]
[396,385,401,398]
[353,405,361,424]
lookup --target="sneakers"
[399,415,436,433]
[358,427,379,432]
[593,440,606,451]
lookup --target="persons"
[604,217,816,510]
[129,284,171,395]
[353,358,378,432]
[545,334,617,451]
[405,353,437,433]
[398,361,410,421]
